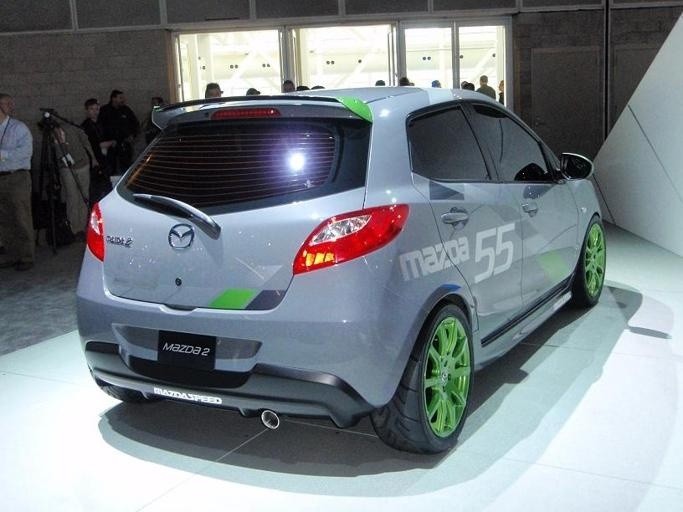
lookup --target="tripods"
[35,129,92,255]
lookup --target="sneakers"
[0,252,19,268]
[16,261,34,270]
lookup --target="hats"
[84,98,100,107]
[246,88,260,94]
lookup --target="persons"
[373,71,505,106]
[0,92,36,271]
[245,88,258,96]
[79,98,116,196]
[101,89,141,176]
[140,98,167,146]
[204,84,222,101]
[279,80,295,94]
[0,93,15,116]
[47,116,96,244]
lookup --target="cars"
[75,86,608,456]
